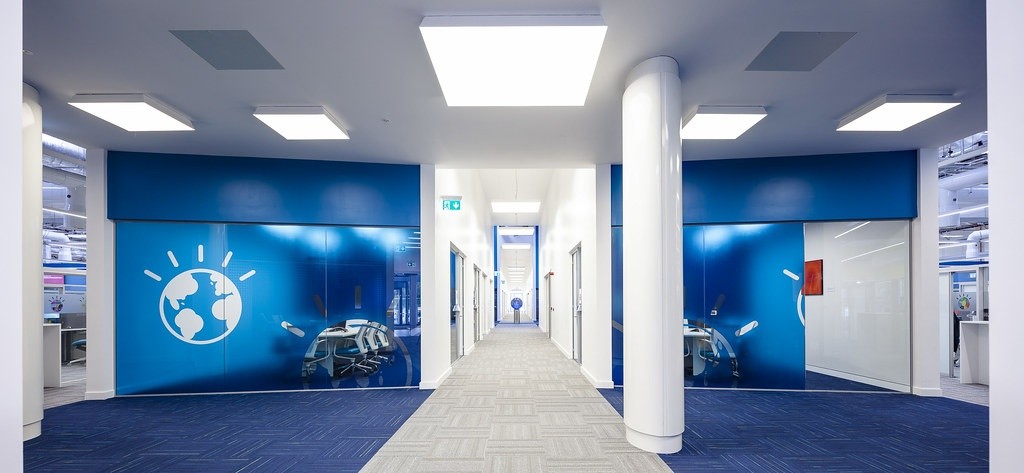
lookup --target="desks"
[43,322,62,389]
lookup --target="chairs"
[67,338,86,366]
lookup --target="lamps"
[252,105,351,142]
[836,93,962,133]
[417,15,608,108]
[681,105,768,140]
[66,91,196,131]
